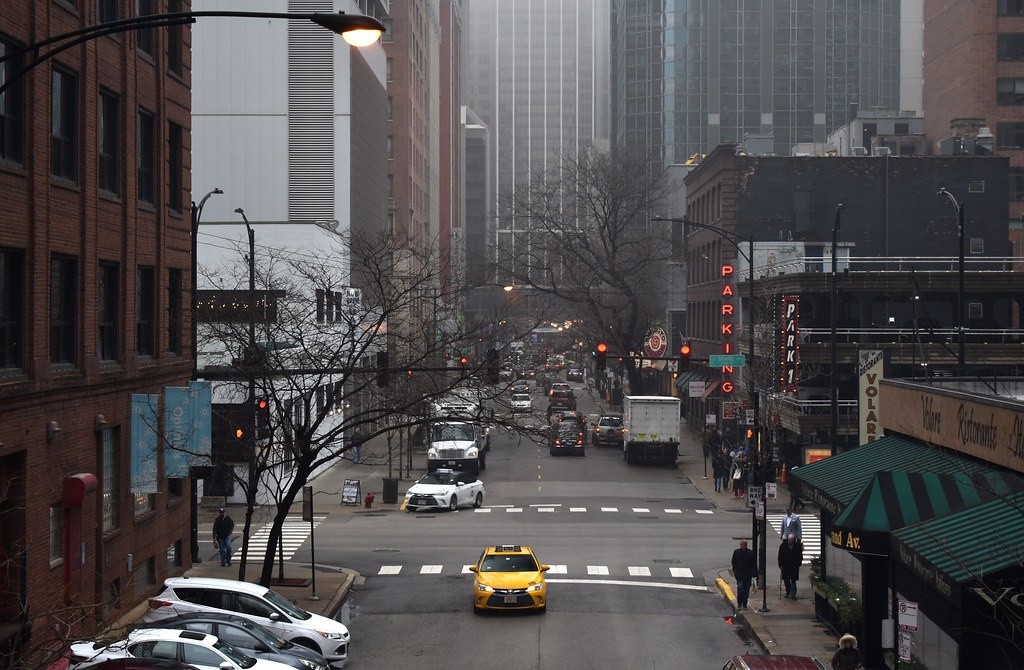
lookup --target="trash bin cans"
[382,478,398,503]
[613,389,623,405]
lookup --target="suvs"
[144,576,351,662]
[591,414,624,446]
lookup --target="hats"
[219,508,225,513]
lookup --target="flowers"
[809,572,856,626]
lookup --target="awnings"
[789,433,1024,607]
[674,368,721,402]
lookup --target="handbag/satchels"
[214,541,219,549]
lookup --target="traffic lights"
[234,426,247,440]
[597,343,606,370]
[680,345,690,372]
[746,428,753,438]
[257,399,270,439]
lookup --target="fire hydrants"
[364,493,374,509]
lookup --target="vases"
[828,597,838,612]
[813,584,827,600]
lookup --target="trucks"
[622,395,682,466]
[428,405,488,475]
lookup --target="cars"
[67,613,330,670]
[524,359,583,413]
[469,545,550,614]
[547,410,588,456]
[510,380,529,395]
[404,468,484,512]
[509,394,534,413]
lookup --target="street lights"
[192,188,223,561]
[653,215,756,490]
[235,208,256,488]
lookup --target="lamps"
[343,401,352,408]
[334,405,342,412]
[95,416,108,430]
[322,406,334,415]
[49,422,60,440]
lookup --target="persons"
[788,478,802,512]
[831,633,864,670]
[712,444,749,499]
[213,508,234,566]
[731,539,756,609]
[780,507,802,542]
[778,533,803,601]
[350,427,364,464]
[587,376,595,393]
[611,377,620,389]
[700,424,723,457]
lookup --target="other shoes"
[738,600,741,608]
[226,560,232,566]
[743,603,747,608]
[784,592,789,598]
[221,561,225,566]
[792,595,797,600]
